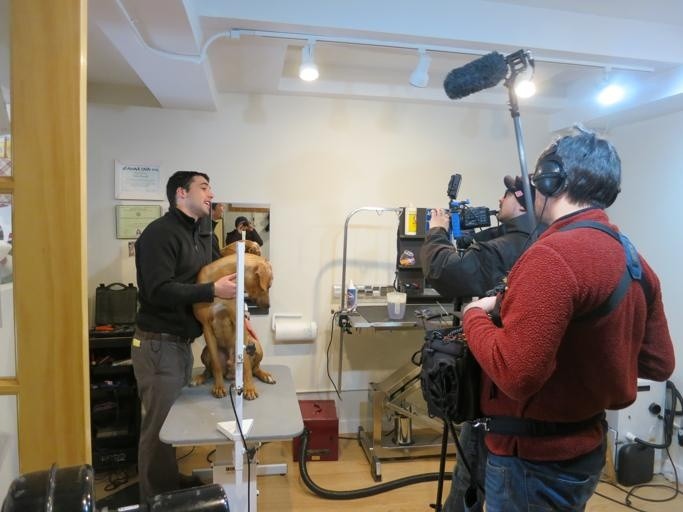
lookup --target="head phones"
[530,136,571,197]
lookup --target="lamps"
[236,28,657,110]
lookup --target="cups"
[386,291,407,321]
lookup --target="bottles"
[394,403,414,447]
[346,280,357,312]
[399,250,414,266]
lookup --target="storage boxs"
[292,396,338,461]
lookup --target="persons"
[211,203,223,261]
[226,216,263,246]
[462,125,675,512]
[130,170,236,512]
[421,174,550,512]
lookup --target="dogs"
[219,240,262,258]
[190,252,276,400]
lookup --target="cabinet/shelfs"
[85,330,144,478]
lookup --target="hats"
[504,173,536,210]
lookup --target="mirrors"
[208,201,270,314]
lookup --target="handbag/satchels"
[420,327,481,424]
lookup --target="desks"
[157,352,304,511]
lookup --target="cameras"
[243,222,248,227]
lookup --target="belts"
[135,327,195,343]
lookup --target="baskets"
[96,282,137,325]
